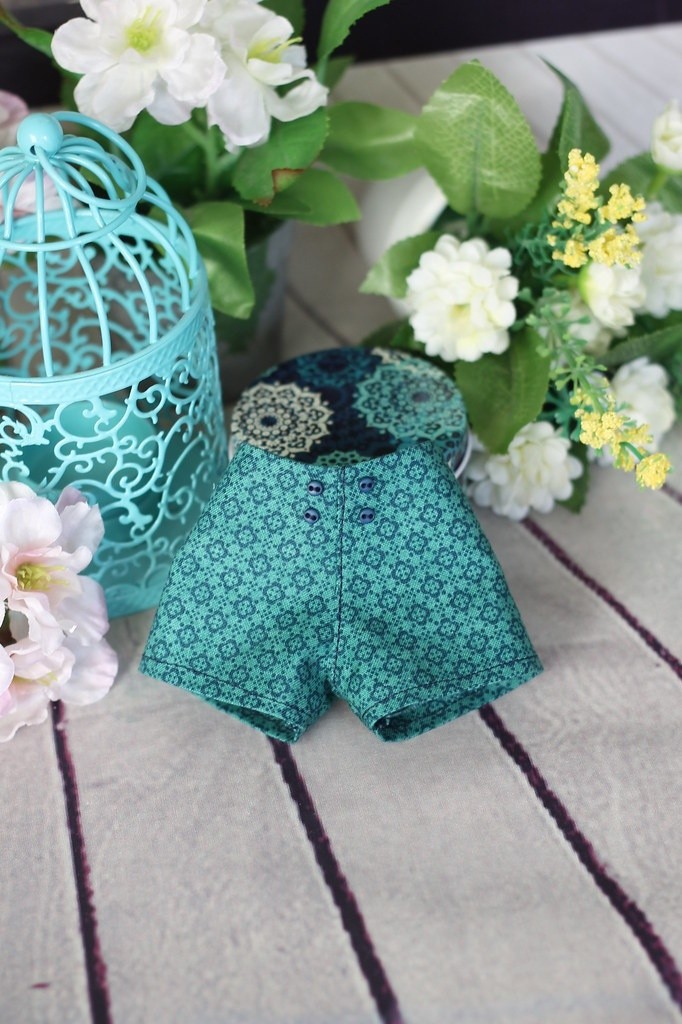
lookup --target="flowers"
[354,55,682,520]
[0,0,431,325]
[0,480,117,749]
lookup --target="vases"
[207,216,296,404]
[348,156,449,320]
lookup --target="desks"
[0,18,682,1024]
